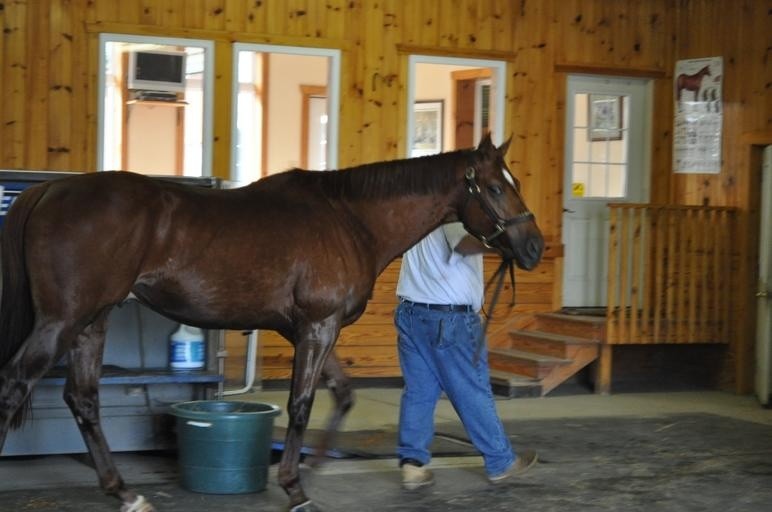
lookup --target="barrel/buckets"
[168,400,281,495]
[169,323,206,370]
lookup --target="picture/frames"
[412,100,443,158]
[587,92,622,141]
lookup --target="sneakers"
[399,464,437,491]
[487,448,539,485]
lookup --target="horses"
[702,73,722,114]
[0,129,547,512]
[676,64,711,102]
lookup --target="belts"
[398,295,475,313]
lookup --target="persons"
[395,220,539,491]
